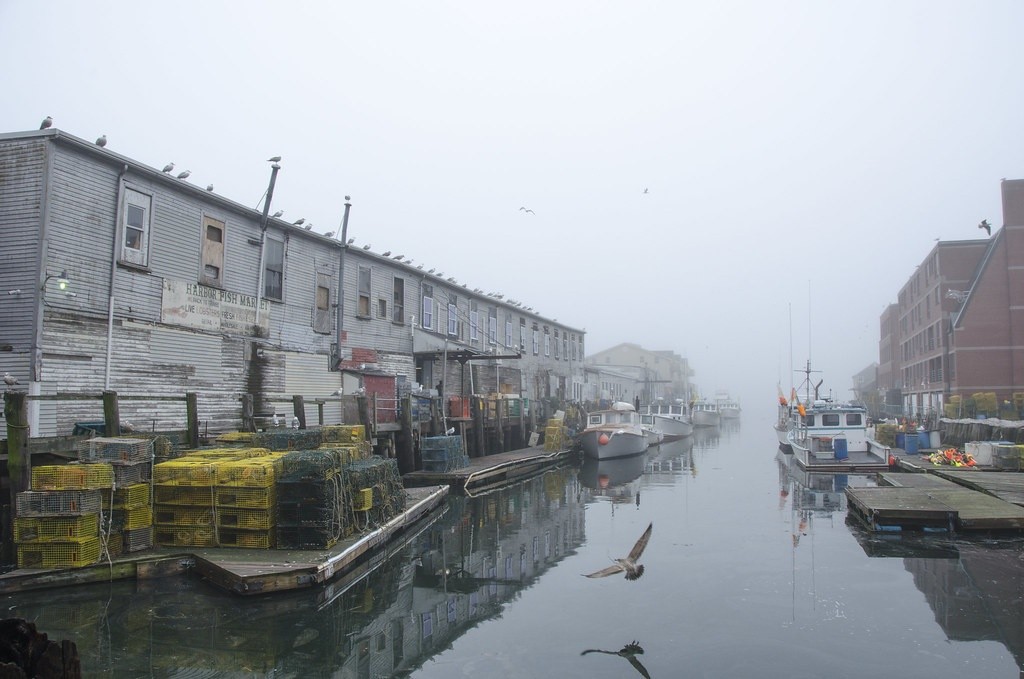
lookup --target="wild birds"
[39,116,53,130]
[580,639,653,679]
[978,219,992,236]
[304,223,313,231]
[580,519,654,581]
[324,230,335,239]
[520,206,535,215]
[95,134,107,147]
[271,385,456,438]
[293,217,306,226]
[644,187,649,193]
[176,169,192,180]
[272,209,285,218]
[346,237,355,245]
[206,183,214,191]
[363,243,586,331]
[162,162,175,174]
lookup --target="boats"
[579,402,649,459]
[774,360,832,446]
[691,401,718,425]
[637,414,664,444]
[787,399,891,472]
[719,403,739,418]
[638,404,693,436]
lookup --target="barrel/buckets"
[834,438,847,458]
[811,437,820,456]
[895,430,941,455]
[819,436,832,452]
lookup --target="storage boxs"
[815,452,835,460]
[528,431,540,447]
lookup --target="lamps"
[41,272,70,300]
